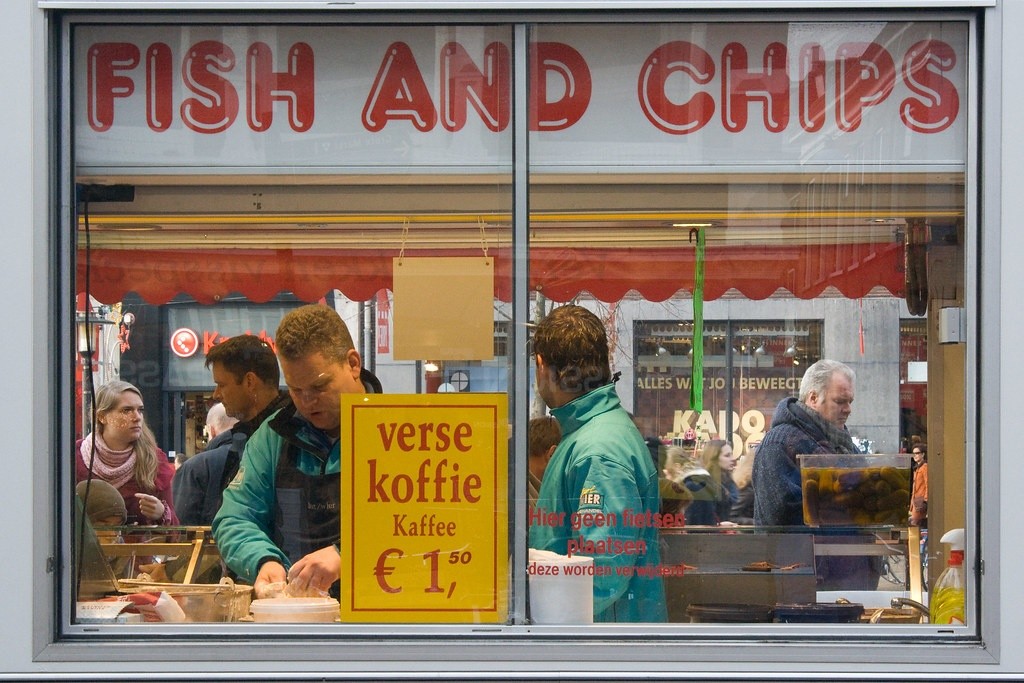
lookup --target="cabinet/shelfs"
[92,526,237,591]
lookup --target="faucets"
[890,595,929,622]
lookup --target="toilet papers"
[527,546,595,625]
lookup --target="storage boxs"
[796,454,914,528]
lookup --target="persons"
[140,334,292,583]
[170,403,242,528]
[75,381,182,580]
[643,438,693,529]
[700,440,742,530]
[908,443,929,512]
[907,497,927,530]
[730,446,759,528]
[750,360,883,626]
[210,306,383,603]
[526,417,561,527]
[662,446,718,527]
[76,480,142,582]
[523,305,669,624]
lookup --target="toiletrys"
[926,528,968,625]
